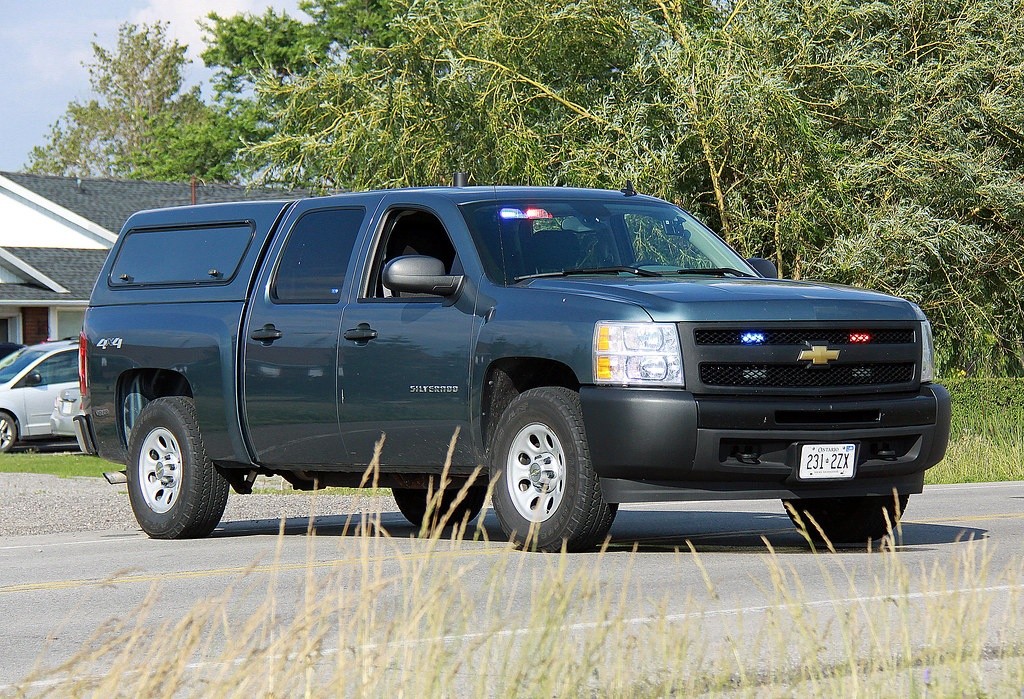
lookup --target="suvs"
[77,173,952,553]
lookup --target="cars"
[0,335,82,455]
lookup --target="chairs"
[394,235,453,298]
[527,229,579,275]
